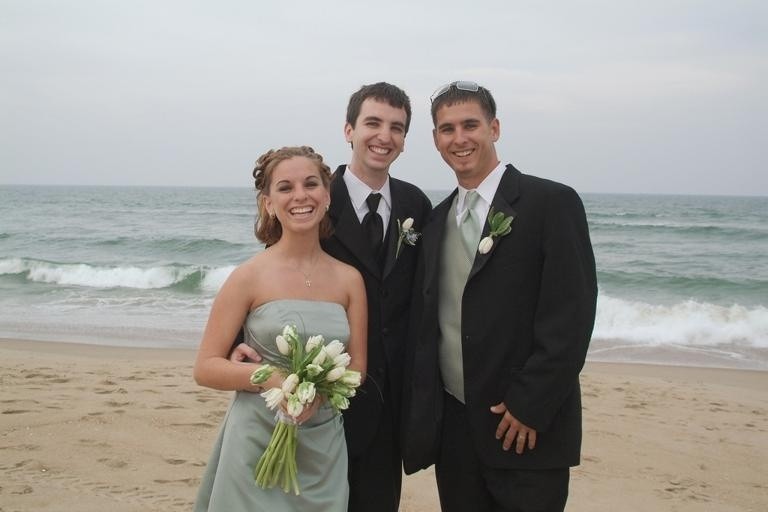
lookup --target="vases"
[244,322,362,497]
[476,203,517,257]
[394,217,424,262]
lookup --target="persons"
[193,147,368,512]
[402,81,598,511]
[228,81,432,512]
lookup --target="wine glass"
[520,433,528,442]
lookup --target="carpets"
[360,193,382,258]
[458,191,480,264]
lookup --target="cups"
[429,80,494,111]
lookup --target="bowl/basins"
[273,243,324,286]
